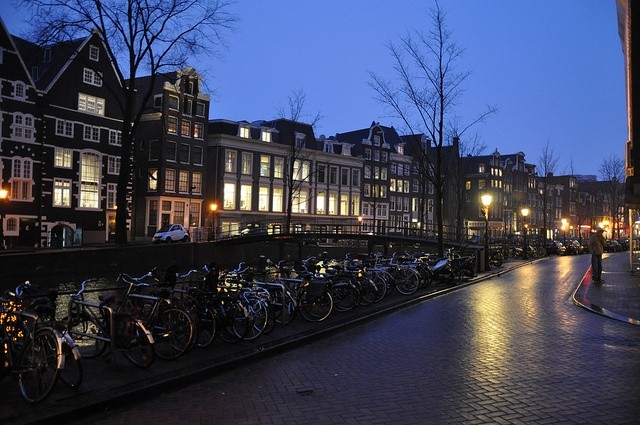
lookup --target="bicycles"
[62,278,155,368]
[0,297,67,402]
[98,274,195,360]
[123,273,200,352]
[8,280,84,390]
[468,234,547,267]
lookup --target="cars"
[582,240,591,254]
[604,240,622,252]
[152,224,191,243]
[618,237,634,251]
[564,240,585,254]
[545,240,563,253]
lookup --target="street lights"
[0,189,8,250]
[603,220,610,239]
[560,218,567,244]
[210,203,217,239]
[358,216,362,233]
[481,194,492,271]
[521,208,529,259]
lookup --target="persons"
[590,228,607,284]
